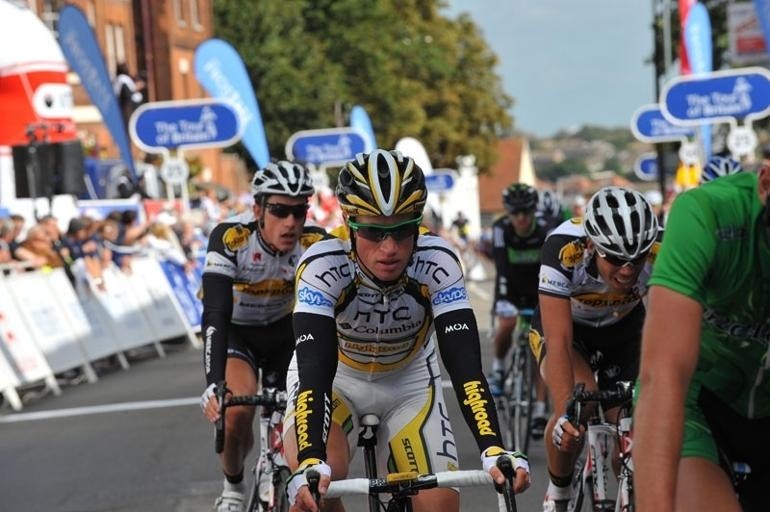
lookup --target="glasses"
[265,203,308,218]
[594,245,650,266]
[506,205,536,214]
[346,213,423,242]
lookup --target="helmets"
[503,183,538,209]
[336,149,427,216]
[584,187,658,259]
[252,161,315,196]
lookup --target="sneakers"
[544,487,574,512]
[531,418,547,439]
[487,370,507,395]
[217,491,245,512]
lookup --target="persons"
[281,148,532,512]
[474,183,553,440]
[451,211,470,245]
[193,186,239,233]
[0,210,196,277]
[537,185,663,512]
[199,159,329,512]
[632,163,770,512]
